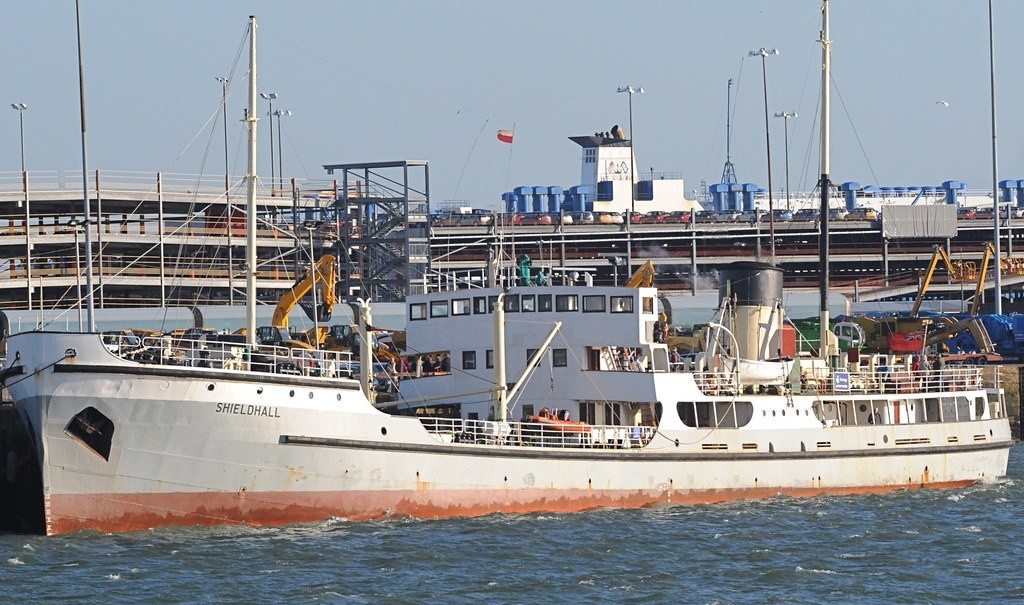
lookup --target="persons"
[108,334,216,370]
[781,347,953,395]
[651,319,682,373]
[395,353,451,381]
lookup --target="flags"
[496,129,514,144]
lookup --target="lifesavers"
[704,371,719,390]
[297,352,314,372]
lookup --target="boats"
[0,0,1024,459]
[5,13,1016,539]
